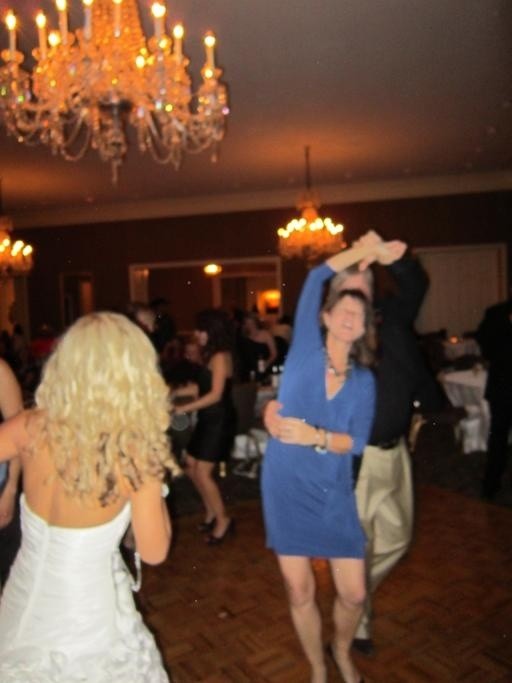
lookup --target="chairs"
[157,337,492,488]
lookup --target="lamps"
[273,144,351,273]
[0,0,231,192]
[0,214,34,279]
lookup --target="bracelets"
[316,427,331,455]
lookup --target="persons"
[1,306,178,683]
[262,235,378,682]
[418,294,512,502]
[131,299,293,549]
[328,228,434,654]
[0,322,38,535]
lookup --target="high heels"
[196,516,234,546]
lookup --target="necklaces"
[326,353,346,380]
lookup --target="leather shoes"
[323,634,373,657]
